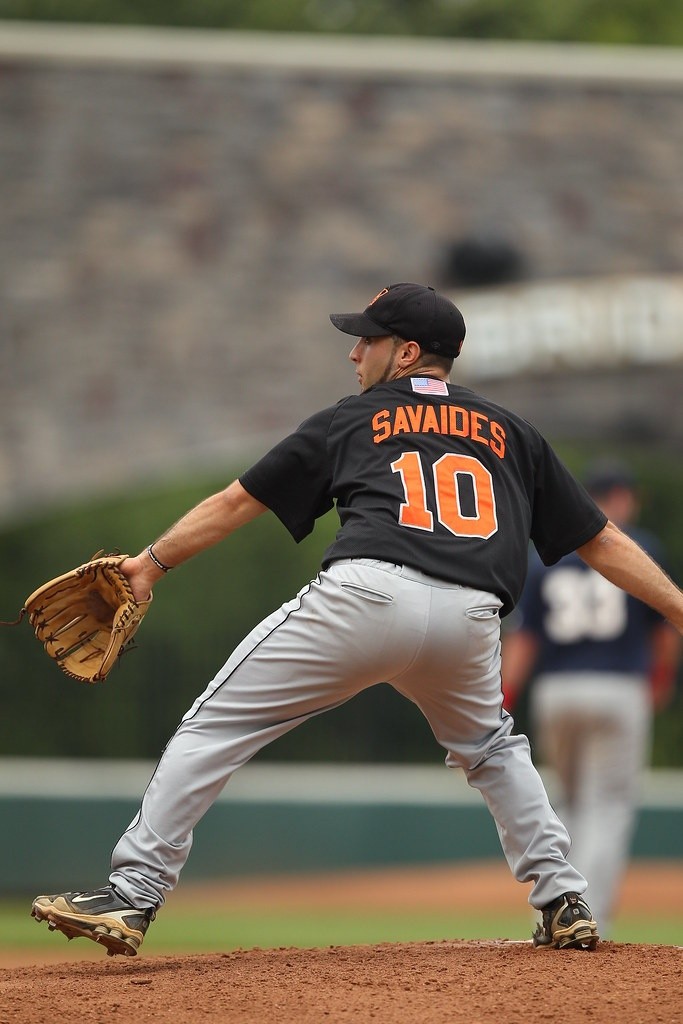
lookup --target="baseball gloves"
[2,549,155,682]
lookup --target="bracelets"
[147,543,174,573]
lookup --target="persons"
[498,467,678,943]
[0,283,683,958]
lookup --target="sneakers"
[532,892,600,952]
[31,886,153,958]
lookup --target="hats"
[329,281,466,359]
[579,468,636,494]
